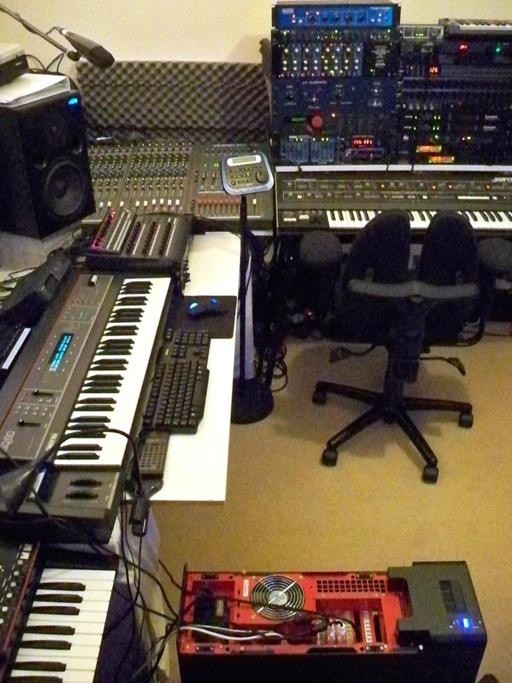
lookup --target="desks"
[126,225,243,505]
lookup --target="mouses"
[186,296,226,317]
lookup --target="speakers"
[1,66,97,241]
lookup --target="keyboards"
[146,325,211,432]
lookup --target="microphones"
[55,25,115,72]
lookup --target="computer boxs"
[175,560,491,679]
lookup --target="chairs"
[298,207,512,486]
